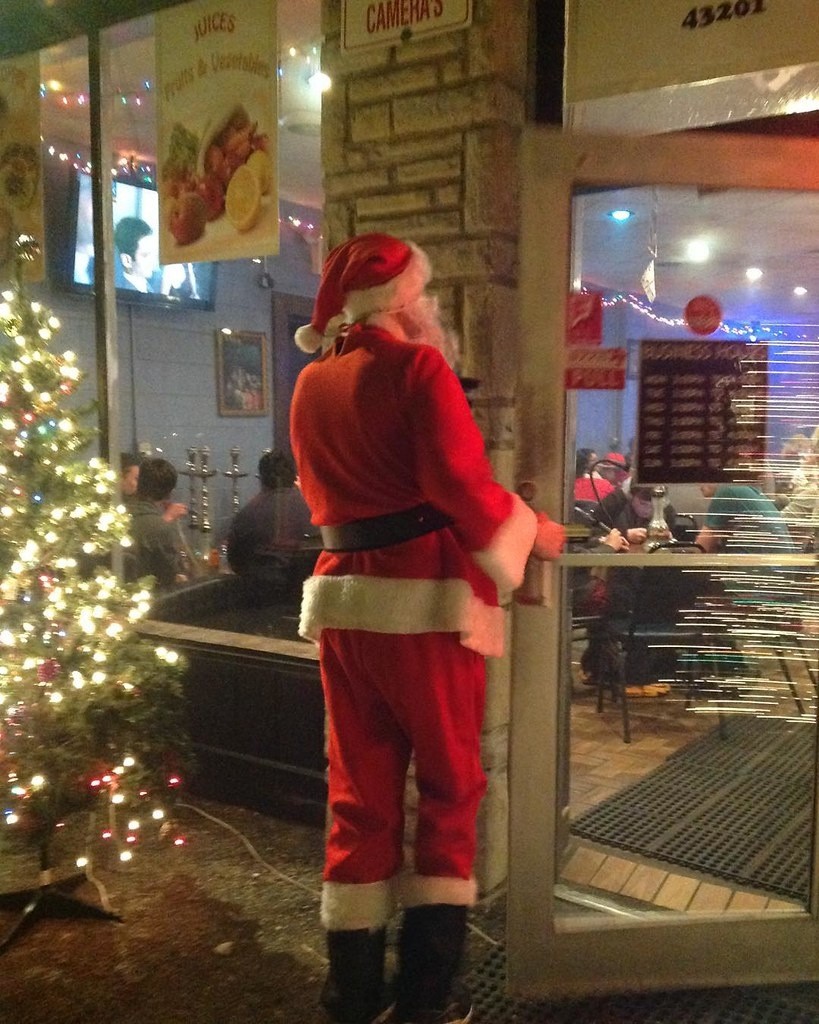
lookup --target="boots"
[396,905,473,1024]
[320,923,395,1024]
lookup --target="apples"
[168,145,231,244]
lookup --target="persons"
[73,173,116,284]
[689,483,796,700]
[116,217,156,295]
[161,263,200,299]
[226,448,315,576]
[124,458,227,597]
[288,232,566,1023]
[121,450,188,523]
[771,427,819,554]
[572,438,677,697]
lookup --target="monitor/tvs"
[61,166,220,312]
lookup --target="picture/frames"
[213,328,274,420]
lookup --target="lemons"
[225,149,273,230]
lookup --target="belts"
[320,502,453,552]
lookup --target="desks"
[556,548,817,737]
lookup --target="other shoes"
[578,669,621,688]
[623,682,662,697]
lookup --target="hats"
[293,233,431,353]
[597,453,626,469]
